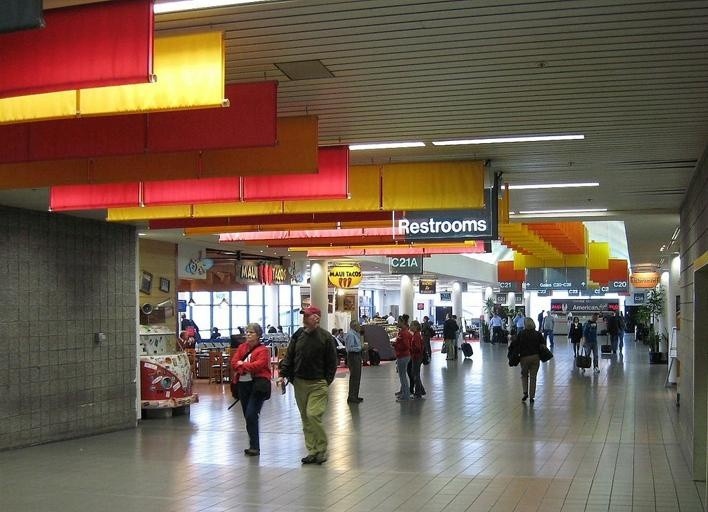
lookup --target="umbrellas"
[347,397,363,403]
[522,396,534,402]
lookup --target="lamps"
[209,351,227,385]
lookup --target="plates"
[364,324,399,360]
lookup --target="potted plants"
[139,270,153,295]
[159,277,170,293]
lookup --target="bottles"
[300,306,321,315]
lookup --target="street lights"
[423,351,431,365]
[254,378,271,400]
[539,344,553,362]
[231,383,238,398]
[576,346,591,368]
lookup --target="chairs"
[302,451,326,463]
[245,449,260,455]
[594,367,600,373]
[395,392,426,402]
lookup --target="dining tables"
[645,288,667,364]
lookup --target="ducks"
[601,345,611,353]
[461,339,473,357]
[369,347,380,365]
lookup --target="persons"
[180,312,201,349]
[275,307,337,464]
[277,325,284,333]
[267,326,277,333]
[508,317,547,405]
[395,371,415,395]
[230,324,272,455]
[210,327,221,340]
[345,319,363,404]
[180,330,194,350]
[489,310,554,349]
[390,313,412,403]
[333,308,462,371]
[408,319,427,399]
[567,310,627,374]
